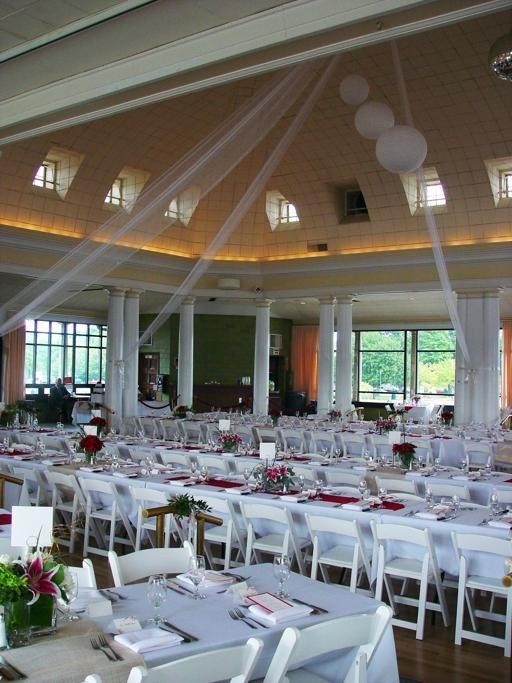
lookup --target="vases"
[412,396,421,402]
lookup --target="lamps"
[217,279,240,289]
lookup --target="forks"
[90,638,116,662]
[233,608,269,629]
[226,609,256,630]
[98,635,124,660]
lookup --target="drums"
[165,621,198,640]
[168,585,184,594]
[158,625,190,643]
[292,598,328,613]
[169,579,193,593]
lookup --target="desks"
[169,384,252,413]
[269,391,281,415]
[69,393,169,423]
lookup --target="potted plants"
[139,331,152,345]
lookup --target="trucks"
[353,101,395,141]
[375,124,428,175]
[338,73,370,106]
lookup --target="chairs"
[0,404,512,683]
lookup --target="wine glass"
[187,555,208,599]
[58,573,80,624]
[146,576,168,624]
[1,407,512,524]
[272,553,291,599]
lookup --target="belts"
[187,555,208,599]
[58,573,80,624]
[146,576,168,624]
[272,553,291,599]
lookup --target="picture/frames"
[169,384,252,413]
[70,395,90,401]
[269,391,281,415]
[139,331,152,345]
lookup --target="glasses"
[217,279,240,289]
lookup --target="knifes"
[158,625,190,643]
[169,579,193,593]
[292,598,328,613]
[165,621,198,640]
[168,585,184,594]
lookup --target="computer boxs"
[254,287,262,293]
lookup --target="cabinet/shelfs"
[138,352,159,398]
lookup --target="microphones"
[57,415,64,423]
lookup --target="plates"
[138,352,159,398]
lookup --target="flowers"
[412,396,421,402]
